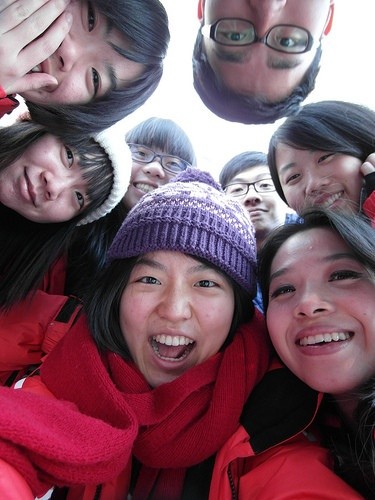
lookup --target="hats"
[106,169,257,299]
[14,111,132,227]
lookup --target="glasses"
[223,178,277,197]
[200,18,320,54]
[126,143,193,174]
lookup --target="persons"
[208,196,375,500]
[191,0,375,316]
[0,0,170,145]
[0,112,134,311]
[0,167,280,500]
[0,116,196,389]
